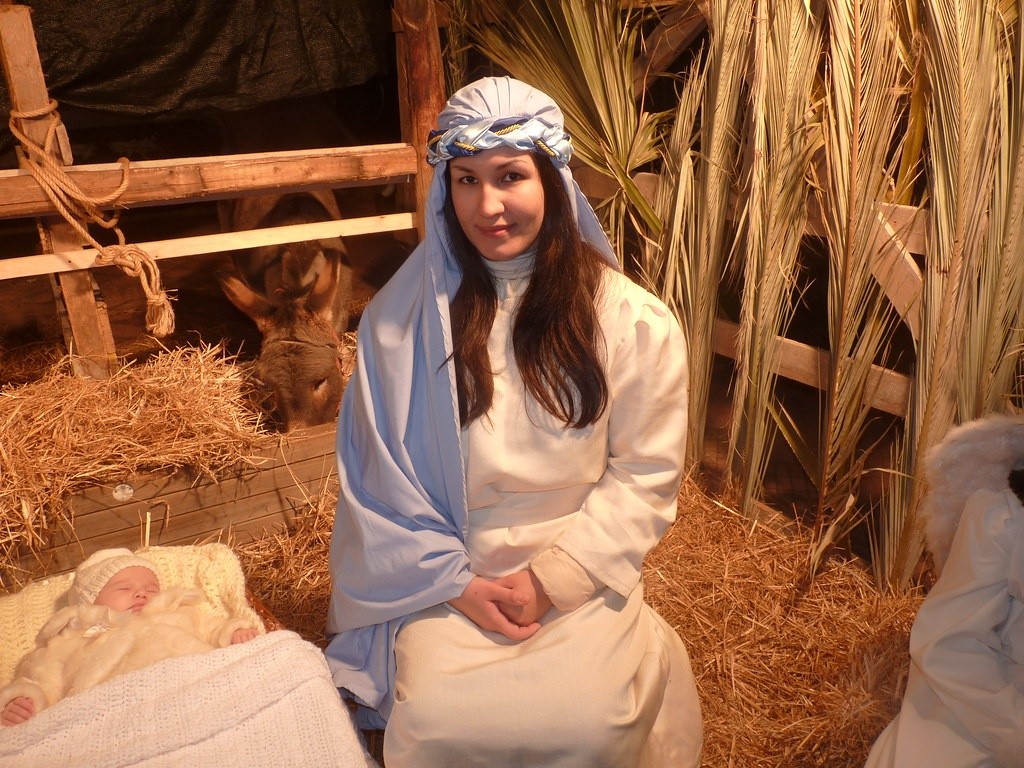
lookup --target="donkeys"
[215,184,353,433]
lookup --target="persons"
[0,548,265,734]
[323,72,702,768]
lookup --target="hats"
[68,548,162,607]
